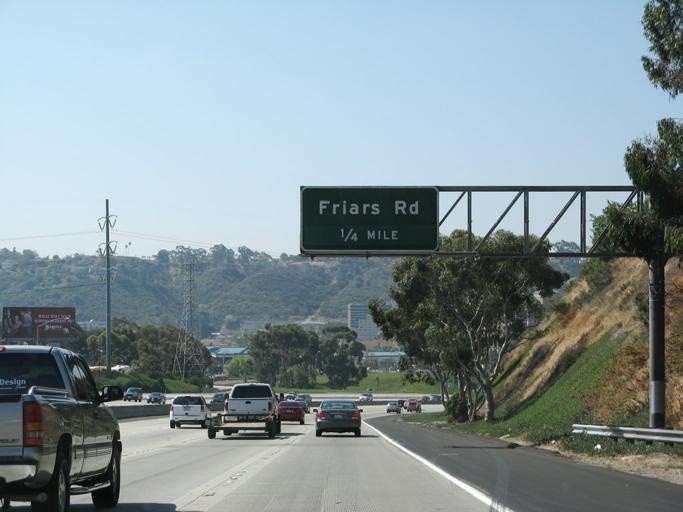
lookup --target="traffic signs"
[298,184,440,257]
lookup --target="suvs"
[124,387,143,402]
[169,395,209,428]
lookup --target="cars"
[398,394,442,412]
[386,402,401,413]
[146,392,166,404]
[207,391,312,425]
[312,399,364,437]
[359,394,373,403]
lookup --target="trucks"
[207,385,280,439]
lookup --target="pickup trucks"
[0,340,124,511]
